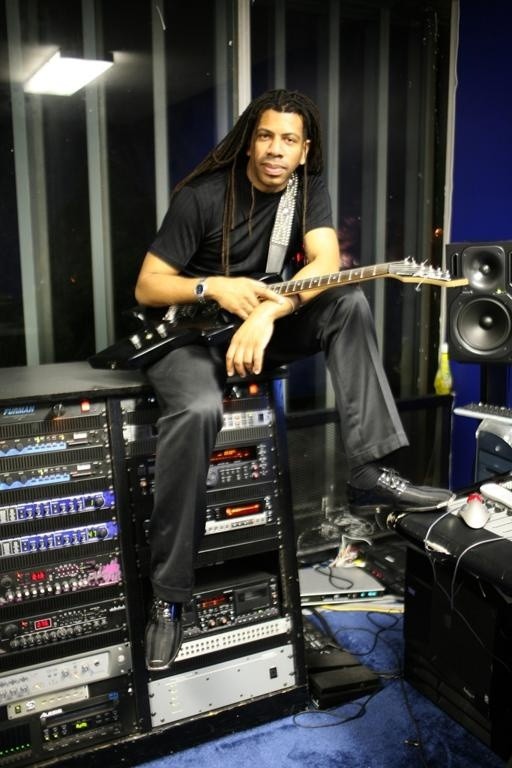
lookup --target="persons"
[135,87,458,673]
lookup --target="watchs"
[195,276,212,306]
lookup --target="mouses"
[458,493,490,529]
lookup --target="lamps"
[21,45,115,101]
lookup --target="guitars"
[90,255,469,371]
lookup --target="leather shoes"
[346,466,457,516]
[144,596,185,673]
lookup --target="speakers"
[446,241,512,364]
